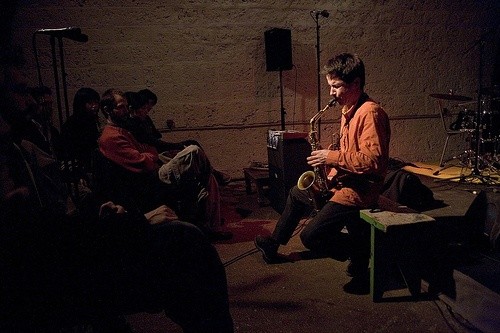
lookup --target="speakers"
[264,28,293,70]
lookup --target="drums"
[456,110,500,153]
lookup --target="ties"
[341,91,370,152]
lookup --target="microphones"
[60,34,88,42]
[37,27,82,36]
[311,10,329,17]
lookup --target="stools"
[242,166,271,207]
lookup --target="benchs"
[358,193,437,303]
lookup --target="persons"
[254,52,391,276]
[0,87,233,333]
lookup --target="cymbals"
[475,85,500,101]
[430,94,473,102]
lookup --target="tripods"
[457,29,497,184]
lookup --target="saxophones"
[298,99,338,215]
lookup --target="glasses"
[116,104,133,112]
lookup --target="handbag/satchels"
[376,168,437,214]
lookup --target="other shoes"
[212,169,232,186]
[193,179,208,202]
[345,251,369,277]
[204,223,233,241]
[254,235,280,264]
